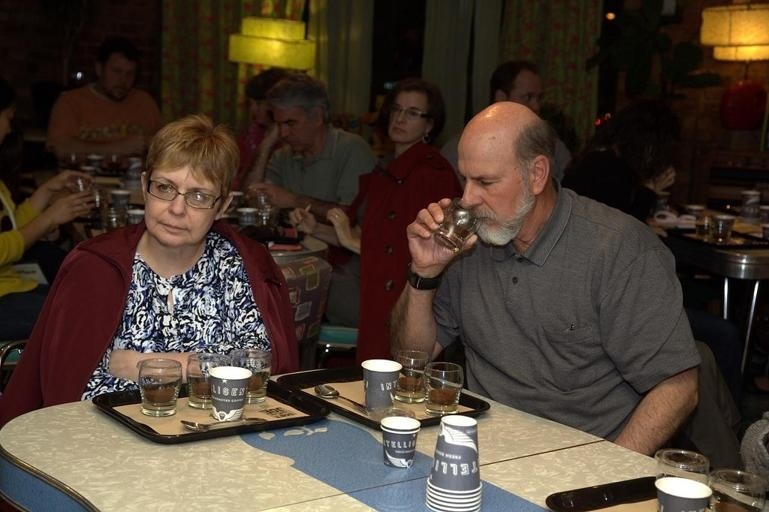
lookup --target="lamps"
[222,13,318,72]
[693,4,769,63]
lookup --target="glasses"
[143,179,223,210]
[390,103,432,120]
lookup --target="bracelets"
[401,266,441,292]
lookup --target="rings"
[335,215,340,219]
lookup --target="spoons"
[315,384,371,415]
[180,416,266,433]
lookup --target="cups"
[424,362,463,417]
[657,191,672,212]
[435,195,482,255]
[138,359,182,417]
[395,349,431,404]
[230,349,271,403]
[654,447,766,511]
[187,354,229,410]
[209,367,253,422]
[682,191,768,244]
[78,155,305,246]
[377,413,483,512]
[361,359,402,415]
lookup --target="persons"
[243,65,297,126]
[284,76,461,326]
[490,62,544,114]
[0,77,96,343]
[248,76,378,230]
[558,101,676,223]
[0,113,301,424]
[389,101,701,461]
[47,41,162,159]
